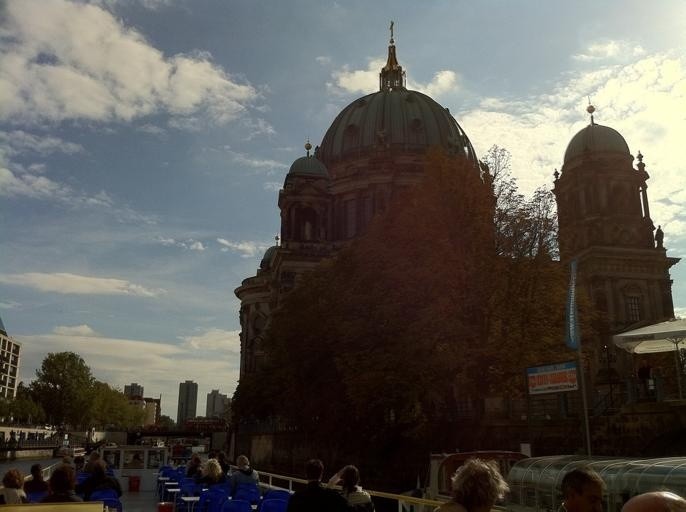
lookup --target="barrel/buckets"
[128,476,140,492]
[157,501,173,512]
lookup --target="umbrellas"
[609,316,686,399]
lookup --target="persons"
[557,465,607,512]
[0,437,262,512]
[325,464,376,511]
[619,489,686,511]
[286,457,353,511]
[432,458,513,512]
